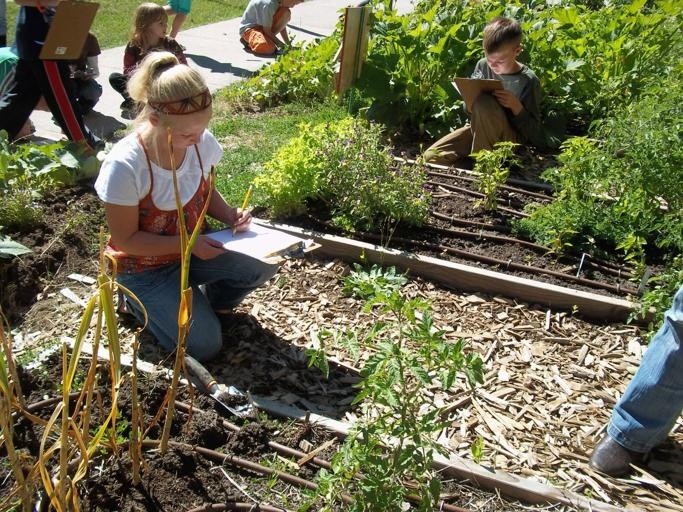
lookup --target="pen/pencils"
[232,185,253,238]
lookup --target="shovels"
[183,353,254,418]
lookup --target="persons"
[422,17,546,167]
[0,0,195,153]
[589,282,681,476]
[238,0,310,56]
[91,48,289,362]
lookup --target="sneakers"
[121,101,133,111]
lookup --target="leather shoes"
[589,434,650,475]
